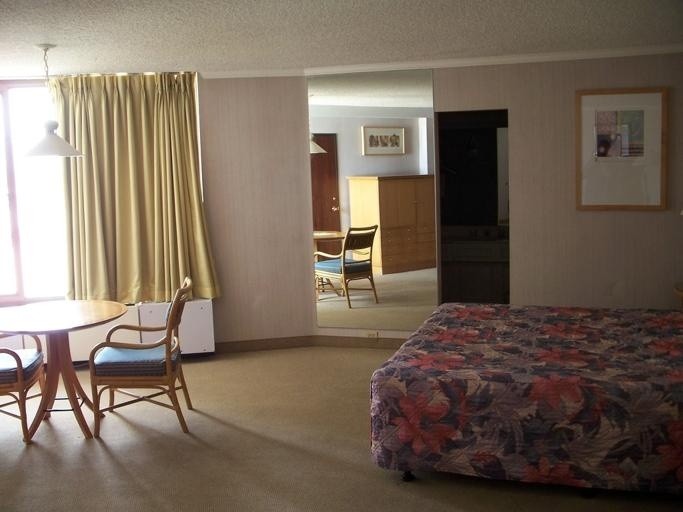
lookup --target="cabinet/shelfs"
[0,296,215,366]
[345,174,436,275]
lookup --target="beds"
[369,302,682,493]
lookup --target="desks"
[313,231,345,293]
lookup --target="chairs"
[0,277,194,445]
[313,224,378,309]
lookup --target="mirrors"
[307,69,442,332]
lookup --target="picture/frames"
[573,87,668,214]
[359,125,407,157]
[495,126,511,227]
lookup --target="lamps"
[23,44,82,160]
[310,134,328,153]
